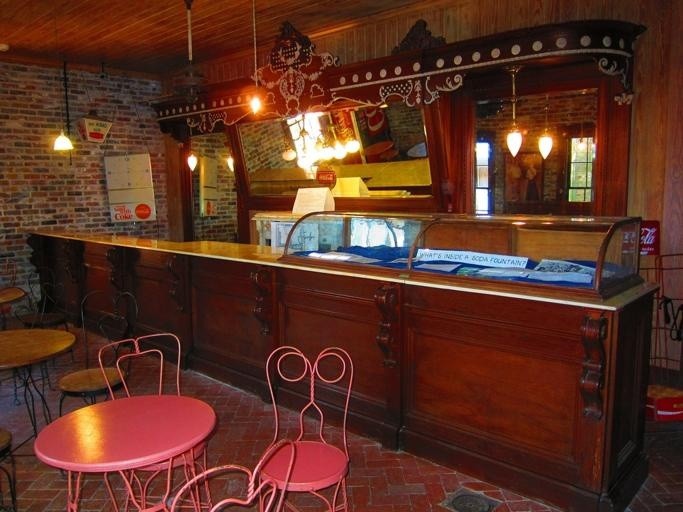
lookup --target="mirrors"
[235,99,433,199]
[187,130,241,243]
[471,86,599,216]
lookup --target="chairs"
[2,258,74,377]
[1,429,18,512]
[97,332,213,512]
[58,290,142,477]
[170,438,295,512]
[258,345,355,511]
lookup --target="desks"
[361,143,396,162]
[406,142,427,160]
[1,328,76,478]
[34,395,218,512]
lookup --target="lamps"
[52,1,75,153]
[537,87,554,161]
[278,110,326,169]
[180,0,204,109]
[186,125,198,172]
[247,0,265,116]
[310,111,360,162]
[227,155,234,171]
[501,63,524,159]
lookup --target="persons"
[523,160,538,201]
[507,159,524,201]
